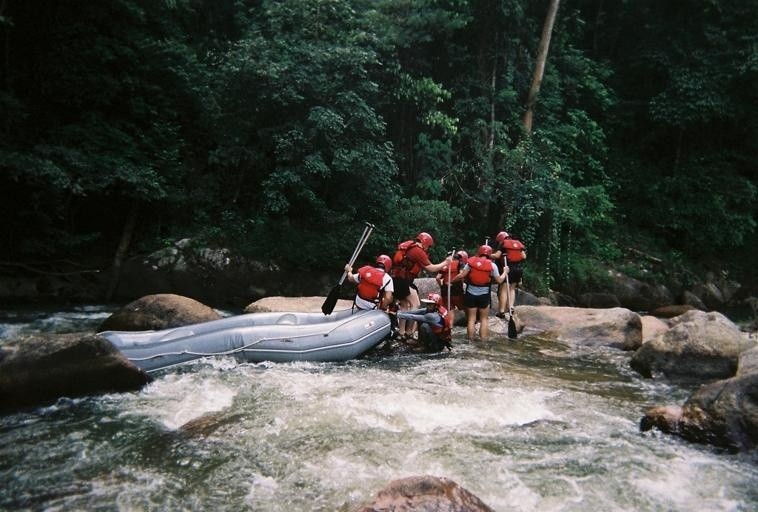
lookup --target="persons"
[391,231,452,339]
[384,293,451,353]
[435,250,469,326]
[345,254,395,310]
[489,231,527,318]
[451,245,510,340]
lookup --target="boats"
[95,309,390,380]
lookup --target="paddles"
[321,221,376,315]
[502,253,517,338]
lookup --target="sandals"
[395,330,416,345]
[495,311,505,318]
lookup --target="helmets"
[417,232,434,250]
[496,232,509,246]
[376,255,393,273]
[458,251,469,263]
[427,294,442,304]
[479,244,493,258]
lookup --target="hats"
[421,299,434,303]
